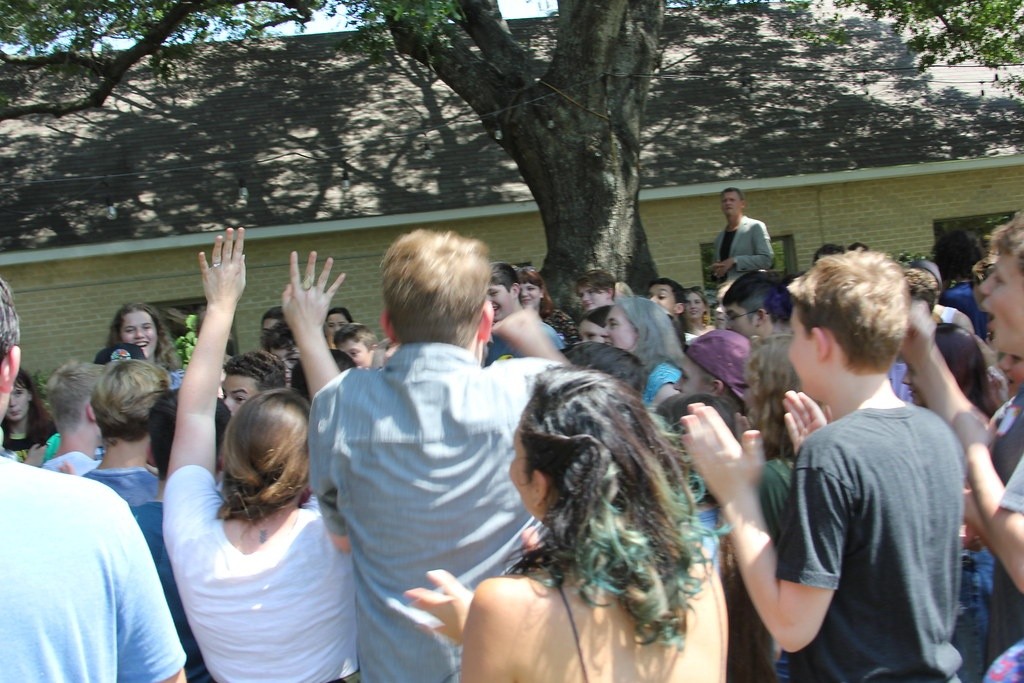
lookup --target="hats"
[911,260,943,291]
[94,343,146,365]
[686,330,750,402]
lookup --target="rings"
[303,275,311,290]
[213,263,220,267]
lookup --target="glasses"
[512,264,537,272]
[682,285,703,292]
[723,311,761,328]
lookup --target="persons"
[710,188,776,281]
[0,223,1024,683]
